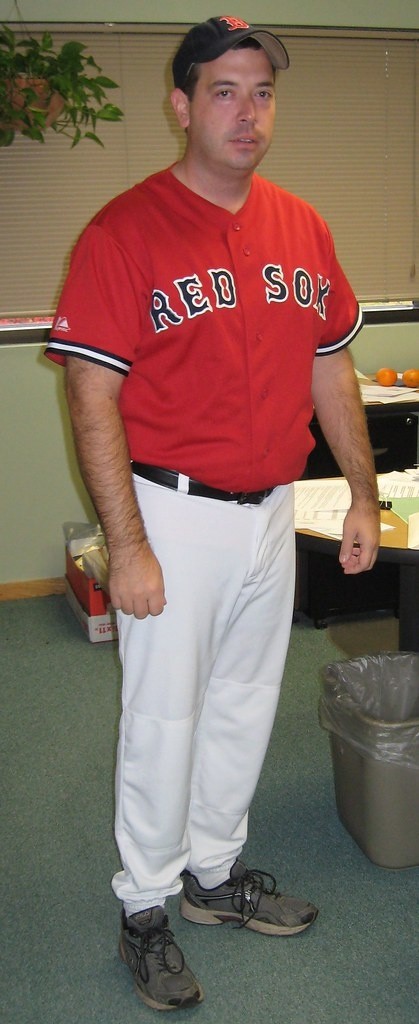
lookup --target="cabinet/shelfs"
[292,371,419,631]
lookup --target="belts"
[131,461,278,505]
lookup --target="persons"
[42,17,385,1013]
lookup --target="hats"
[172,15,289,90]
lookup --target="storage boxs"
[65,537,119,643]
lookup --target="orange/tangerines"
[401,369,419,387]
[376,368,397,386]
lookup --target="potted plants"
[0,24,125,149]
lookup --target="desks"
[295,473,419,654]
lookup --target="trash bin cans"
[320,650,419,869]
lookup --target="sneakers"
[120,905,204,1011]
[180,856,319,935]
